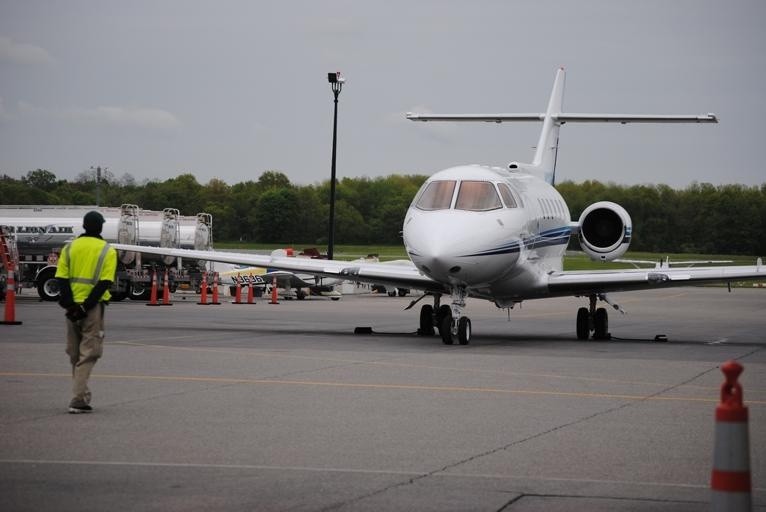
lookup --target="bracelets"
[78,302,87,313]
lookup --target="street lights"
[89,166,101,206]
[325,69,348,261]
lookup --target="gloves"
[63,301,88,321]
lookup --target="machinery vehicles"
[0,201,219,303]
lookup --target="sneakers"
[68,404,94,413]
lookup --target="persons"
[52,210,122,416]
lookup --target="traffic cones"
[144,268,281,307]
[0,261,25,326]
[705,356,757,512]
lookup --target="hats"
[84,211,105,228]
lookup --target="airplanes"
[69,64,766,349]
[205,247,380,302]
